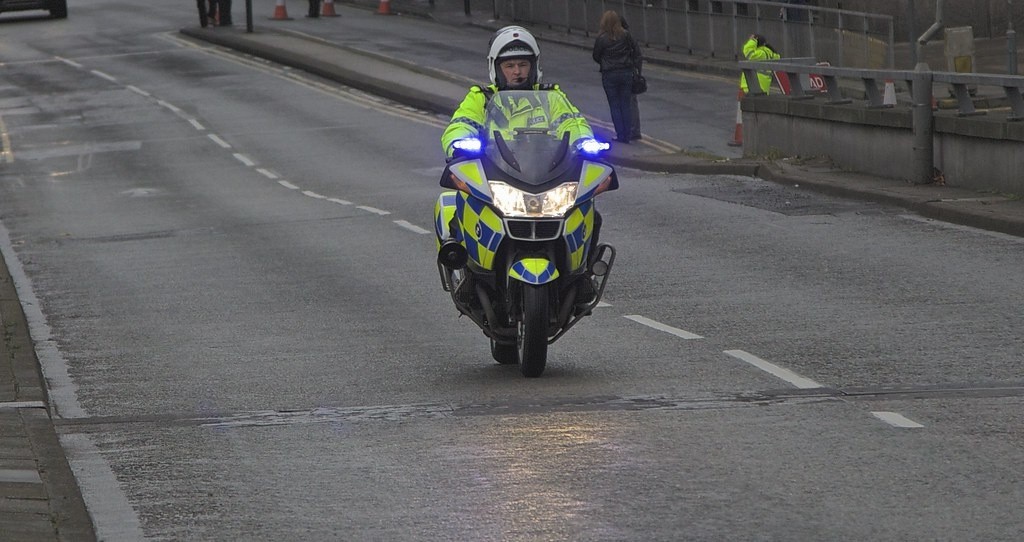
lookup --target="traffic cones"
[729,89,747,146]
[272,0,294,20]
[320,0,341,16]
[882,78,898,105]
[376,0,394,15]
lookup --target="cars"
[0,0,68,20]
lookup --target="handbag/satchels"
[633,73,647,94]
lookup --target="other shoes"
[613,136,630,144]
[728,140,741,147]
[631,135,641,139]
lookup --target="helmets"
[486,26,544,87]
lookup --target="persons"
[305,0,321,18]
[593,10,643,142]
[439,24,619,316]
[197,0,217,28]
[740,34,781,96]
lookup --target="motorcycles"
[432,89,617,377]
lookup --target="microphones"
[518,77,531,82]
